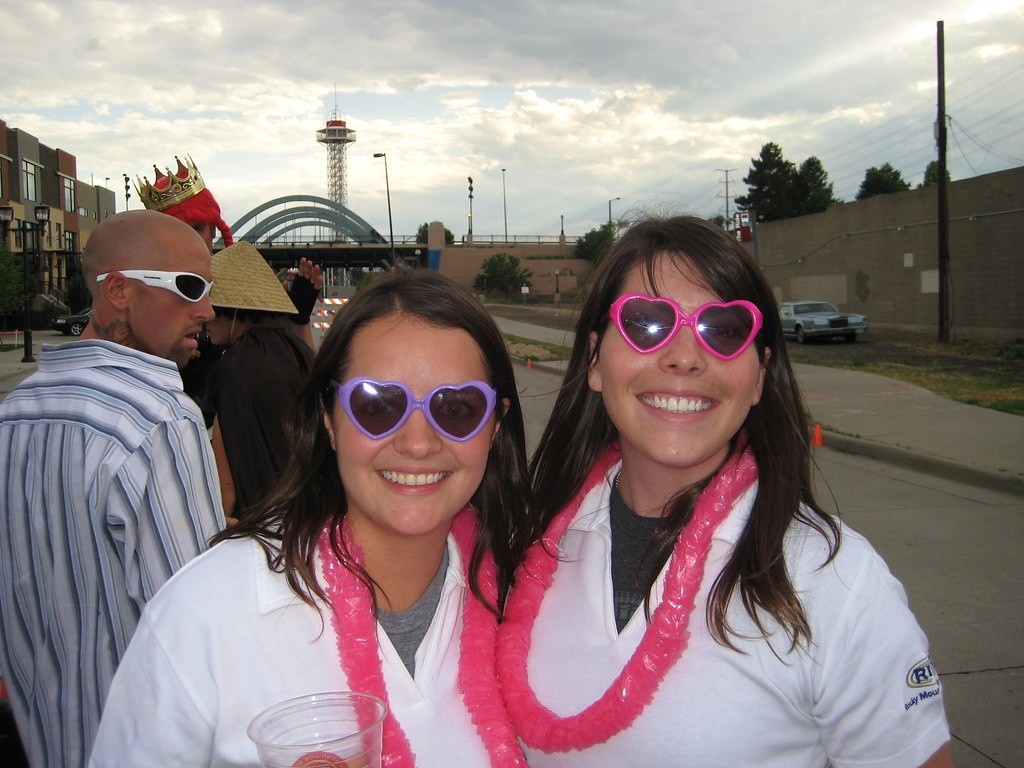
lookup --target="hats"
[128,153,233,248]
[210,240,300,315]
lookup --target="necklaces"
[318,506,528,767]
[494,436,764,754]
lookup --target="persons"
[498,207,953,768]
[80,268,532,768]
[0,209,326,768]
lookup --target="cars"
[778,300,868,344]
[50,308,94,336]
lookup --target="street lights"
[374,152,395,264]
[502,168,507,243]
[0,206,51,362]
[609,197,620,230]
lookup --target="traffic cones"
[812,424,824,446]
[526,358,532,368]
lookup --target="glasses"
[603,292,765,362]
[328,378,498,444]
[95,270,215,304]
